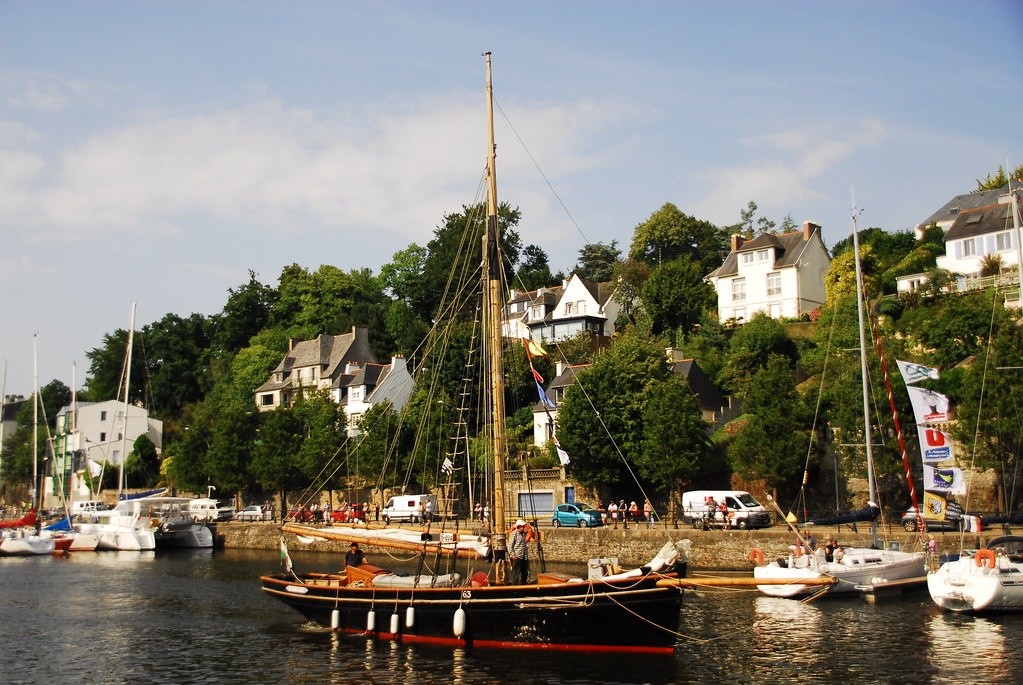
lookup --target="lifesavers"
[510,521,533,543]
[975,548,996,568]
[750,548,764,566]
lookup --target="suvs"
[900,503,956,532]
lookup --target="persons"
[800,529,819,556]
[508,519,530,585]
[474,501,492,527]
[416,499,432,525]
[343,500,380,527]
[927,534,937,574]
[704,496,731,530]
[599,499,657,529]
[262,500,274,520]
[344,542,369,567]
[298,500,331,525]
[824,538,845,563]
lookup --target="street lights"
[207,484,216,522]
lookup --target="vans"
[682,490,771,529]
[190,498,233,522]
[380,494,438,525]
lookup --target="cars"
[289,506,319,521]
[236,505,275,523]
[552,502,602,528]
[330,503,366,522]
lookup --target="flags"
[281,543,293,572]
[440,457,455,477]
[964,515,982,534]
[896,359,968,523]
[514,319,570,467]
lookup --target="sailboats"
[260,51,694,664]
[1,300,214,556]
[924,156,1023,612]
[749,183,941,598]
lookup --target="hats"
[620,500,624,502]
[631,501,636,505]
[514,519,526,527]
